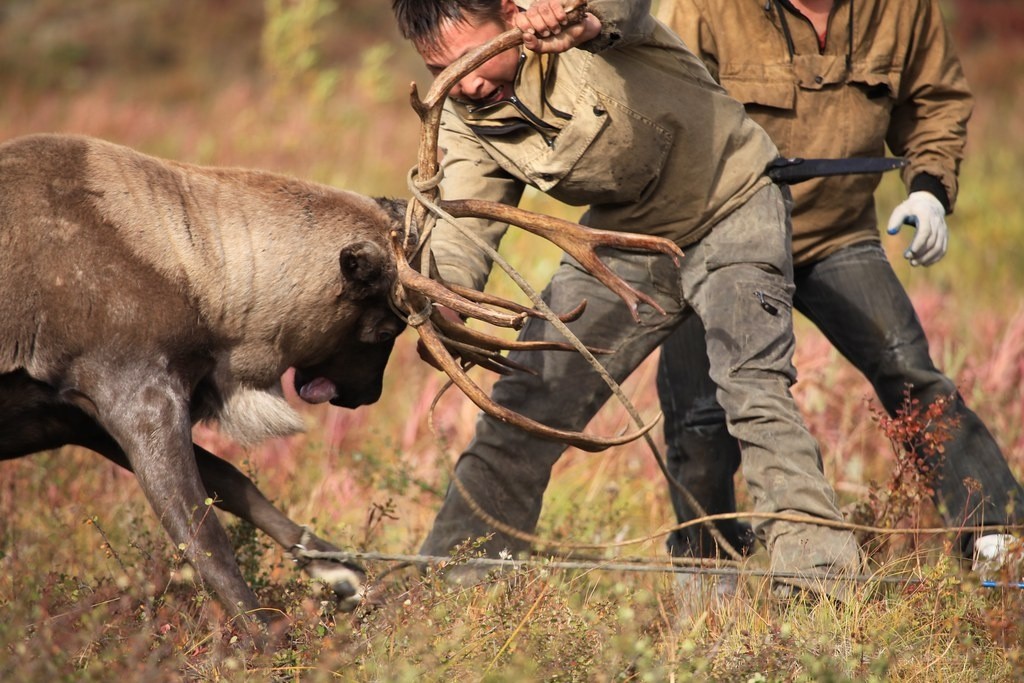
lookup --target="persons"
[644,0,1024,594]
[380,0,880,620]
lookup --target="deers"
[1,1,686,666]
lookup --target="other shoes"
[968,533,1023,585]
[668,569,738,629]
[749,582,837,624]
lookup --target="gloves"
[886,190,948,268]
